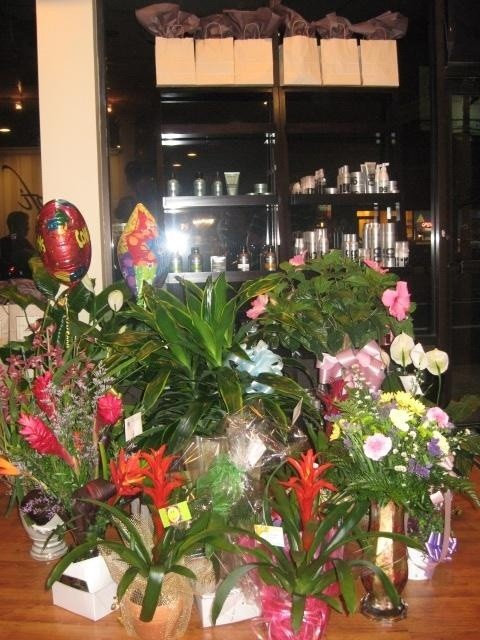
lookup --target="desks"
[0,446,480,640]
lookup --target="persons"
[0,210,38,281]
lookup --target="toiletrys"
[224,172,241,197]
[380,162,391,193]
[364,161,376,193]
[375,163,383,193]
[360,164,367,194]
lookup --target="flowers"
[94,391,128,434]
[18,371,75,457]
[379,279,413,321]
[338,395,452,503]
[243,291,275,319]
[7,324,79,375]
[115,446,169,540]
[279,452,340,522]
[387,334,452,380]
[286,252,391,276]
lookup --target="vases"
[12,482,67,558]
[120,573,193,640]
[251,582,331,640]
[361,491,409,621]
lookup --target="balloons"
[35,199,92,289]
[115,201,175,307]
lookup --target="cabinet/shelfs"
[157,88,407,264]
